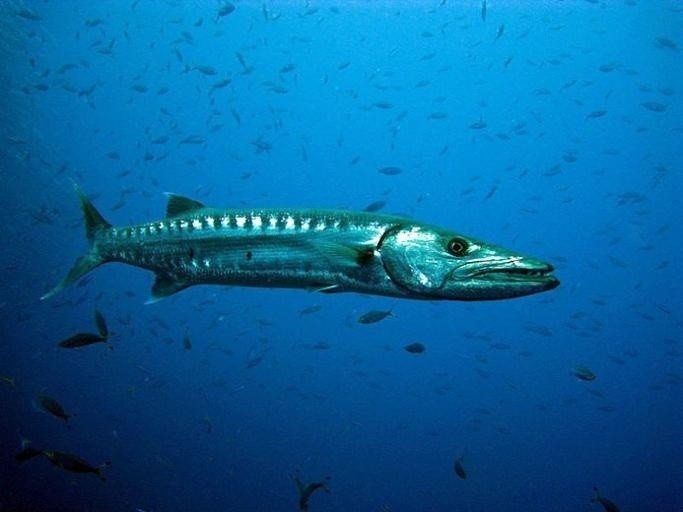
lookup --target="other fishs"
[0,0,683,511]
